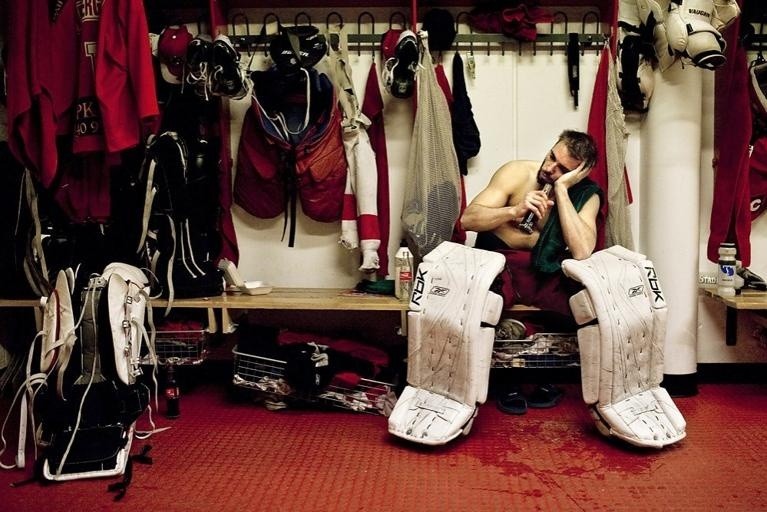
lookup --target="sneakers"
[182,33,250,101]
[494,374,563,416]
[382,30,421,100]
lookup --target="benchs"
[704,286,766,346]
[0,287,543,337]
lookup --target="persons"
[386,131,689,450]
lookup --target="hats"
[156,21,196,85]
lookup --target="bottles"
[164,365,181,418]
[716,242,736,297]
[394,238,415,301]
